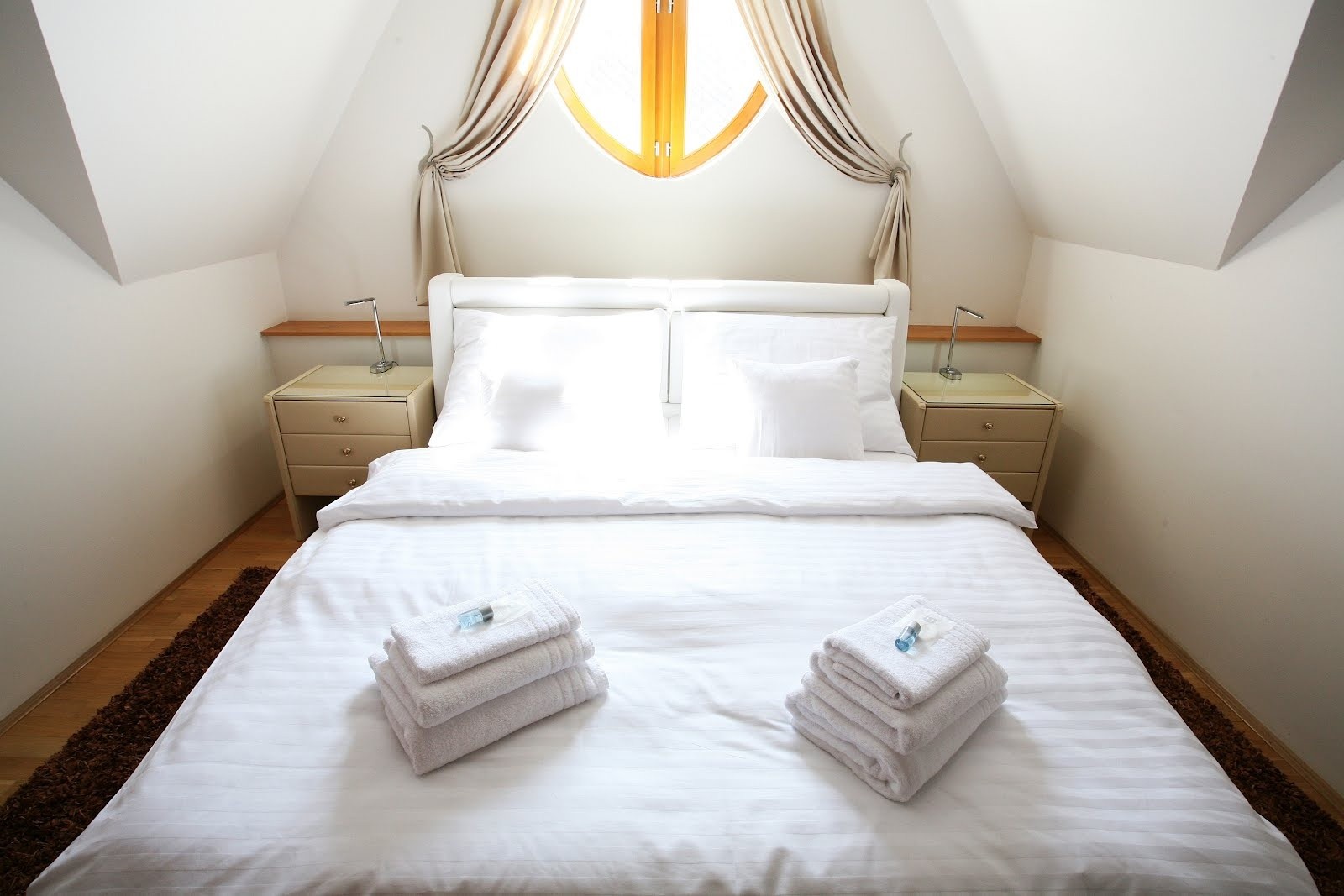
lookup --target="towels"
[389,576,582,686]
[801,648,1009,756]
[367,627,595,729]
[379,656,610,776]
[822,594,991,710]
[784,685,1008,804]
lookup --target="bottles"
[457,603,494,630]
[894,620,921,651]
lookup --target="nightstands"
[900,372,1065,538]
[262,365,437,542]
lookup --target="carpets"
[0,565,279,896]
[1056,568,1344,896]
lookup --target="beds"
[28,275,1325,896]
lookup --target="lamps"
[344,297,393,372]
[939,304,983,380]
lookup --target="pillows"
[426,306,919,462]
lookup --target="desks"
[261,319,431,337]
[907,324,1042,343]
